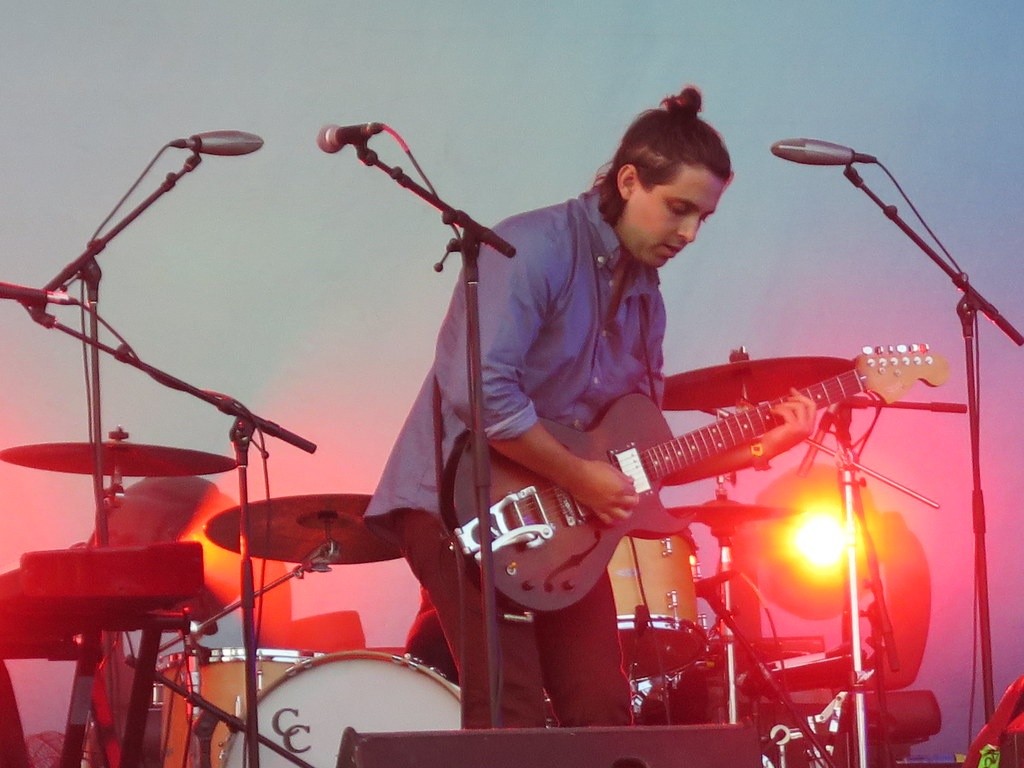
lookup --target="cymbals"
[205,494,404,565]
[663,501,785,524]
[664,357,851,410]
[0,443,235,478]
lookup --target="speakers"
[333,724,764,768]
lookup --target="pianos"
[4,540,205,658]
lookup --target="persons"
[362,85,820,731]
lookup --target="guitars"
[443,344,950,613]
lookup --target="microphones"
[317,123,385,154]
[770,138,877,166]
[170,129,265,157]
[0,282,78,305]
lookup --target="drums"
[154,648,323,768]
[607,528,698,680]
[222,650,463,768]
